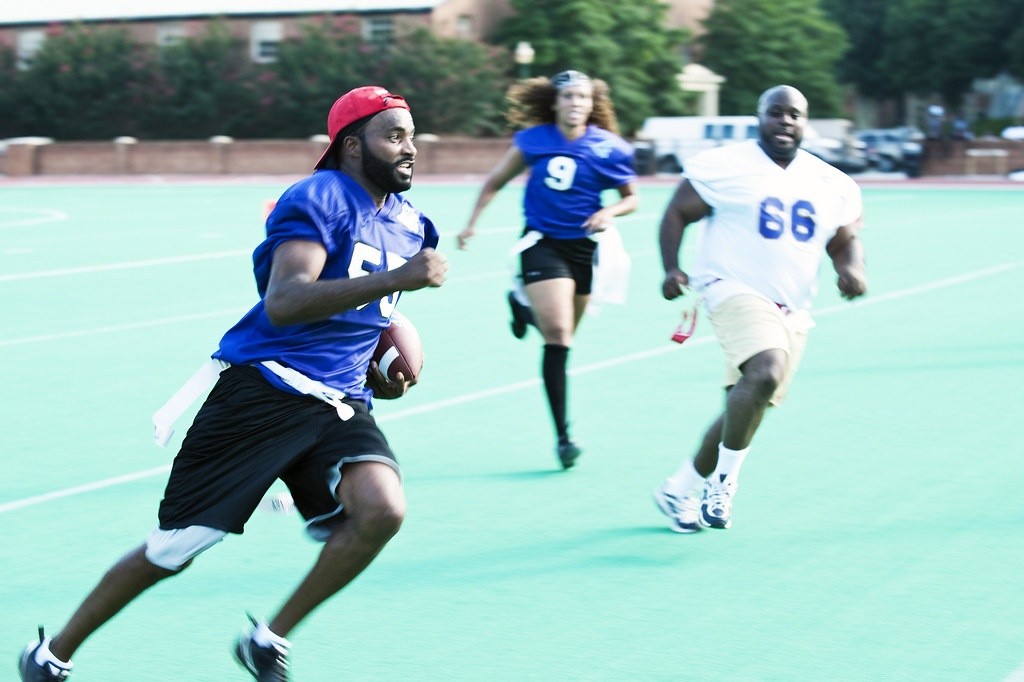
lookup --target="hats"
[313,87,411,171]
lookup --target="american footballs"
[371,312,422,381]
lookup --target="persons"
[456,69,637,467]
[17,85,441,682]
[658,82,869,535]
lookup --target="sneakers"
[700,473,738,530]
[507,291,528,339]
[556,441,583,470]
[234,612,291,682]
[17,626,75,682]
[654,476,703,534]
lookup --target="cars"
[626,110,973,178]
